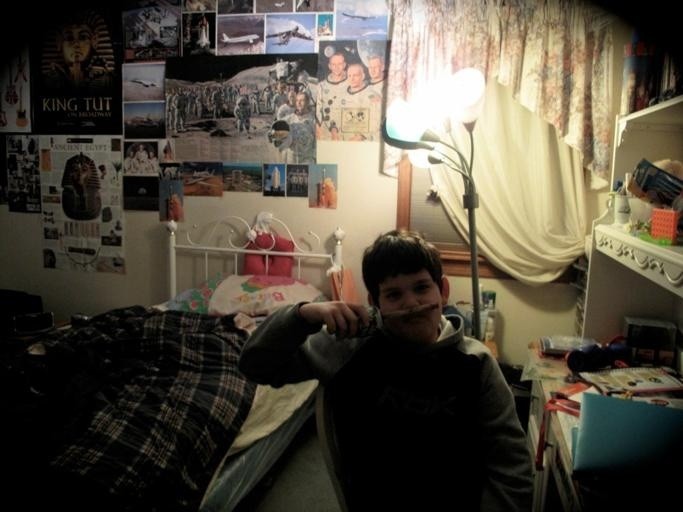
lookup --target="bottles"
[477,289,505,357]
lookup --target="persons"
[0,0,389,177]
[237,226,537,511]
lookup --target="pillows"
[207,274,322,322]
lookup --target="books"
[577,366,682,395]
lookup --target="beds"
[2,209,346,512]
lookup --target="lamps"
[384,64,531,344]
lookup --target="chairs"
[313,380,353,512]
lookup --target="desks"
[524,92,682,511]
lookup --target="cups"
[627,196,661,224]
[605,195,630,225]
